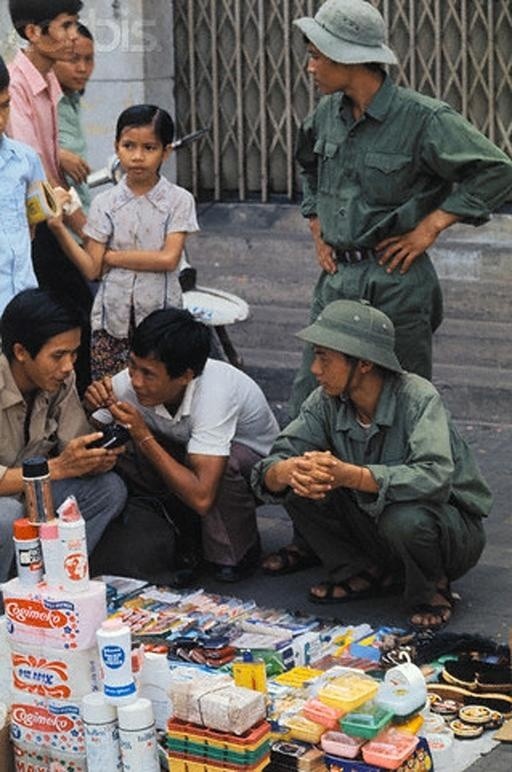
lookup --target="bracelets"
[138,435,154,448]
[356,467,363,490]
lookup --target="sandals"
[254,544,313,577]
[407,577,459,632]
[306,566,397,607]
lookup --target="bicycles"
[85,125,252,369]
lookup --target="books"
[25,179,61,225]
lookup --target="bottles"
[57,495,90,592]
[22,456,54,525]
[82,692,124,772]
[38,524,67,586]
[117,698,161,772]
[95,617,138,706]
[12,520,45,585]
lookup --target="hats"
[293,298,410,376]
[293,0,400,66]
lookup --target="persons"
[3,0,91,386]
[51,20,100,296]
[260,0,512,576]
[79,307,280,589]
[0,55,47,352]
[0,288,128,614]
[47,103,199,431]
[251,298,496,632]
